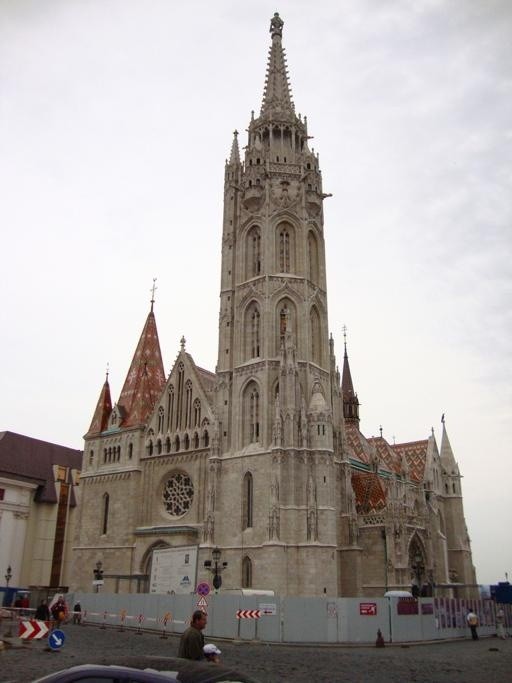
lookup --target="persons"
[177,610,207,662]
[494,605,506,640]
[14,593,81,629]
[203,644,221,664]
[466,608,479,640]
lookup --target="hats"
[203,644,221,655]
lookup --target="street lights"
[204,546,228,595]
[413,548,426,597]
[93,560,104,591]
[5,565,12,587]
